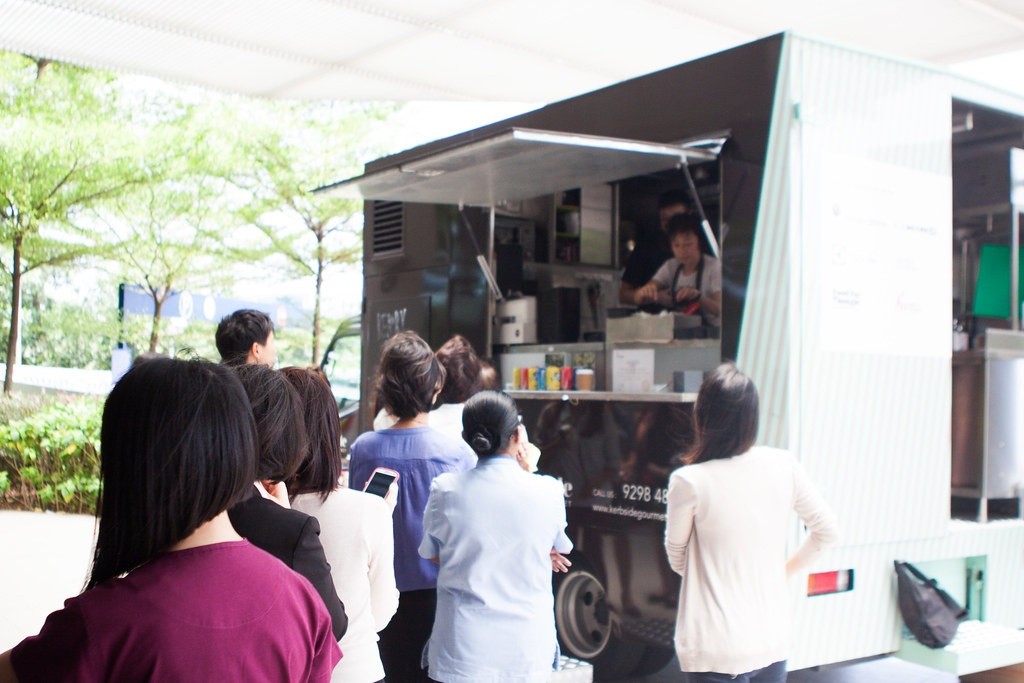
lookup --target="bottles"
[513,366,574,390]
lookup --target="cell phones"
[360,467,400,499]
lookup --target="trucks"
[322,31,1024,683]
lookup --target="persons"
[631,211,723,338]
[617,185,695,302]
[535,395,694,628]
[661,363,838,683]
[0,310,575,683]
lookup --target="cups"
[576,368,595,390]
